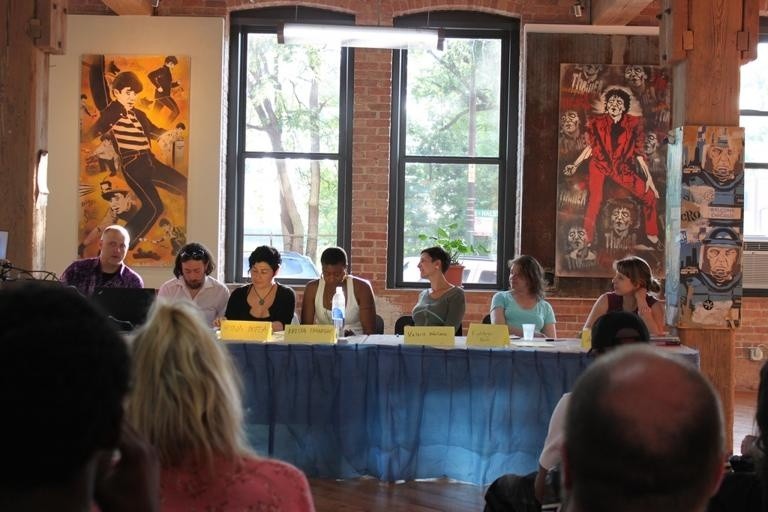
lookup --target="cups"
[522,324,535,341]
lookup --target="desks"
[208,325,699,492]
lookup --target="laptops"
[94,287,155,330]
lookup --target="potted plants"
[418,222,491,288]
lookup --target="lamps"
[274,0,447,53]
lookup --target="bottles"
[332,287,346,340]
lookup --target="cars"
[238,247,319,280]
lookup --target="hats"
[585,311,649,359]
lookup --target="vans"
[400,255,496,286]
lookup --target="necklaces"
[251,283,277,306]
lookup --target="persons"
[2,273,168,512]
[490,252,556,337]
[146,242,229,329]
[54,226,145,294]
[118,297,318,511]
[741,431,765,459]
[580,254,666,334]
[211,245,296,334]
[412,247,466,337]
[479,311,652,510]
[558,347,729,507]
[300,245,376,335]
